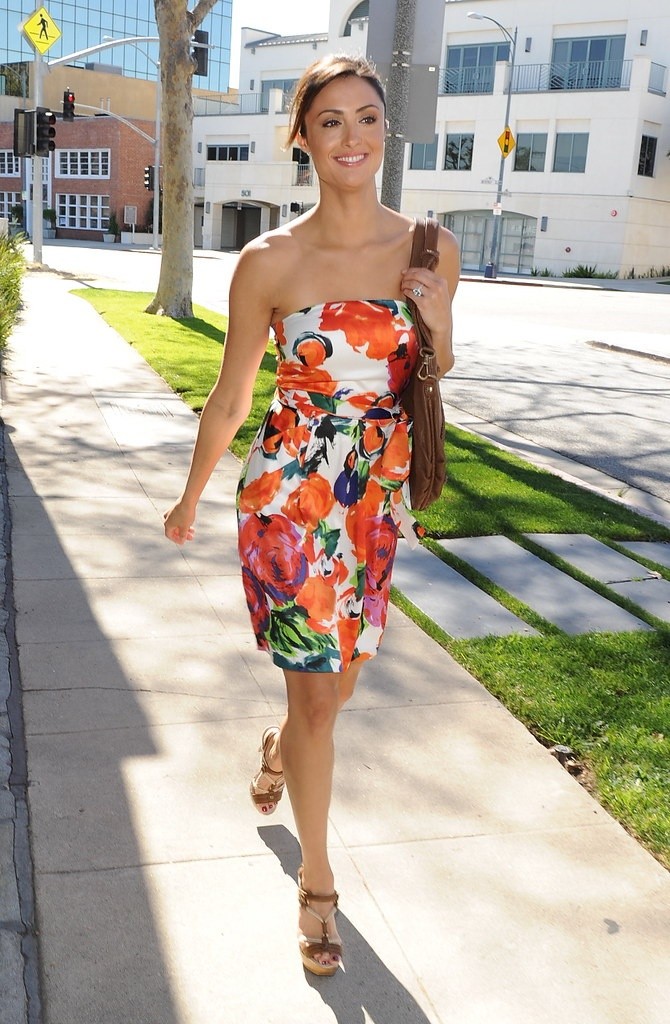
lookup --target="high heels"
[297,863,342,976]
[250,726,285,815]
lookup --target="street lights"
[102,34,163,250]
[466,12,518,278]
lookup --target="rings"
[412,284,425,297]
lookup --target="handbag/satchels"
[409,218,446,510]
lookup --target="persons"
[164,58,460,978]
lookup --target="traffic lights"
[63,91,75,122]
[143,165,154,193]
[36,107,56,157]
